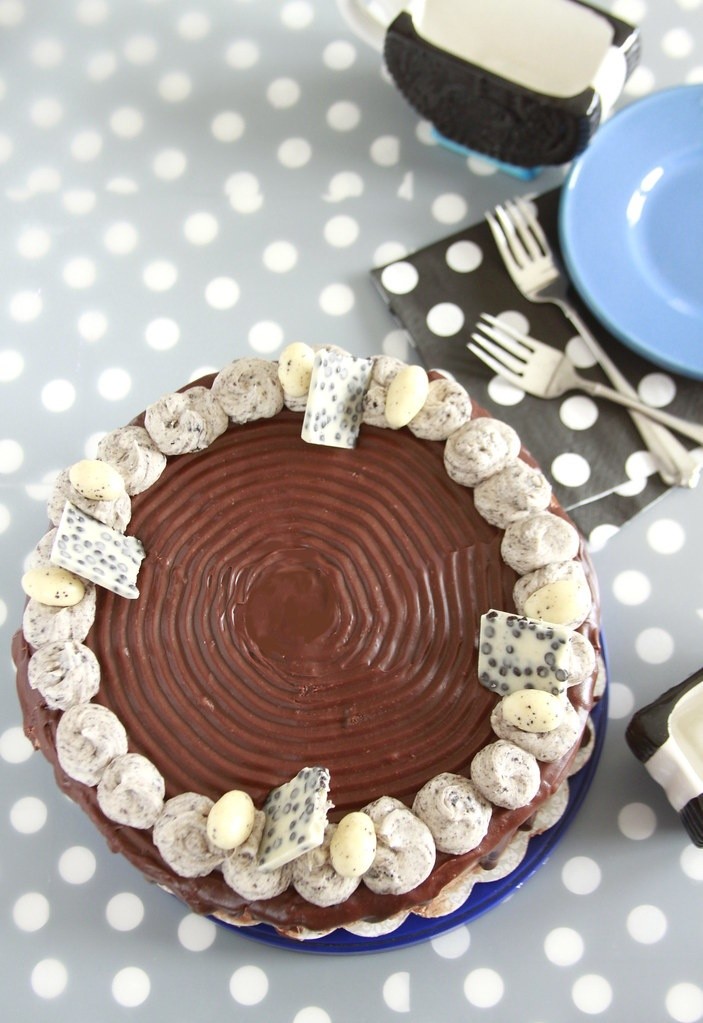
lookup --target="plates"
[9,362,608,959]
[558,83,702,382]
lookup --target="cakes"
[13,341,611,942]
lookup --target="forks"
[485,196,699,489]
[467,312,703,441]
